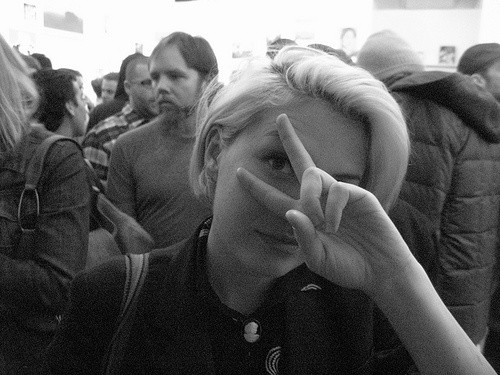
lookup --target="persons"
[354,30,500,375]
[47,44,498,375]
[0,32,90,375]
[33,38,352,197]
[457,43,500,104]
[107,32,219,252]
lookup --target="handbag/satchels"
[18,134,156,331]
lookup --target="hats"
[356,28,424,82]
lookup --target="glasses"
[130,79,153,90]
